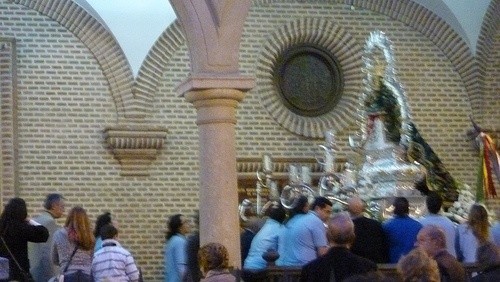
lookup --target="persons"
[162,193,500,282]
[0,192,139,282]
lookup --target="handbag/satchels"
[64,270,96,282]
[19,270,35,282]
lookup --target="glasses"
[181,219,187,226]
